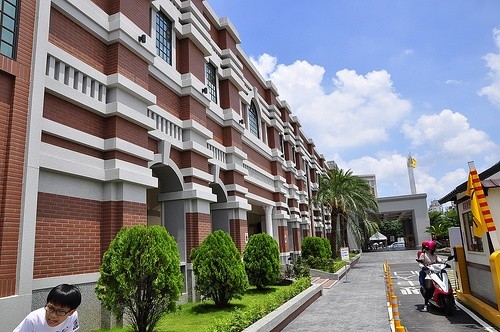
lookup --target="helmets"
[422,241,437,253]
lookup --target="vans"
[391,242,405,248]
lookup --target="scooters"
[413,256,456,315]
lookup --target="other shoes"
[420,285,424,288]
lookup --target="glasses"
[45,306,73,317]
[425,246,429,251]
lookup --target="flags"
[465,170,496,238]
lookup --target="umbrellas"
[369,231,387,244]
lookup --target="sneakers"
[423,306,427,311]
[454,305,460,310]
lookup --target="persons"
[13,284,81,332]
[418,241,446,312]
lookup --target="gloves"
[422,267,429,270]
[444,263,451,268]
